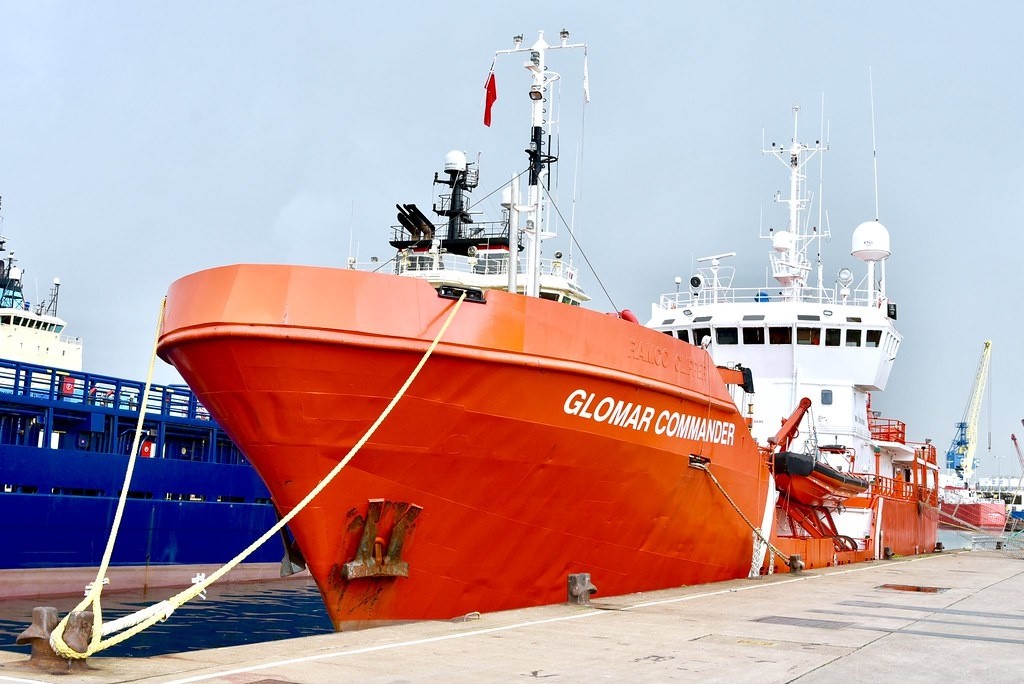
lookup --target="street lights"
[995,455,1006,504]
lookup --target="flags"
[484,60,496,127]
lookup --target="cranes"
[942,338,993,495]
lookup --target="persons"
[894,468,903,498]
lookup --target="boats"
[937,482,1024,533]
[156,25,941,633]
[0,195,327,607]
[768,451,870,509]
